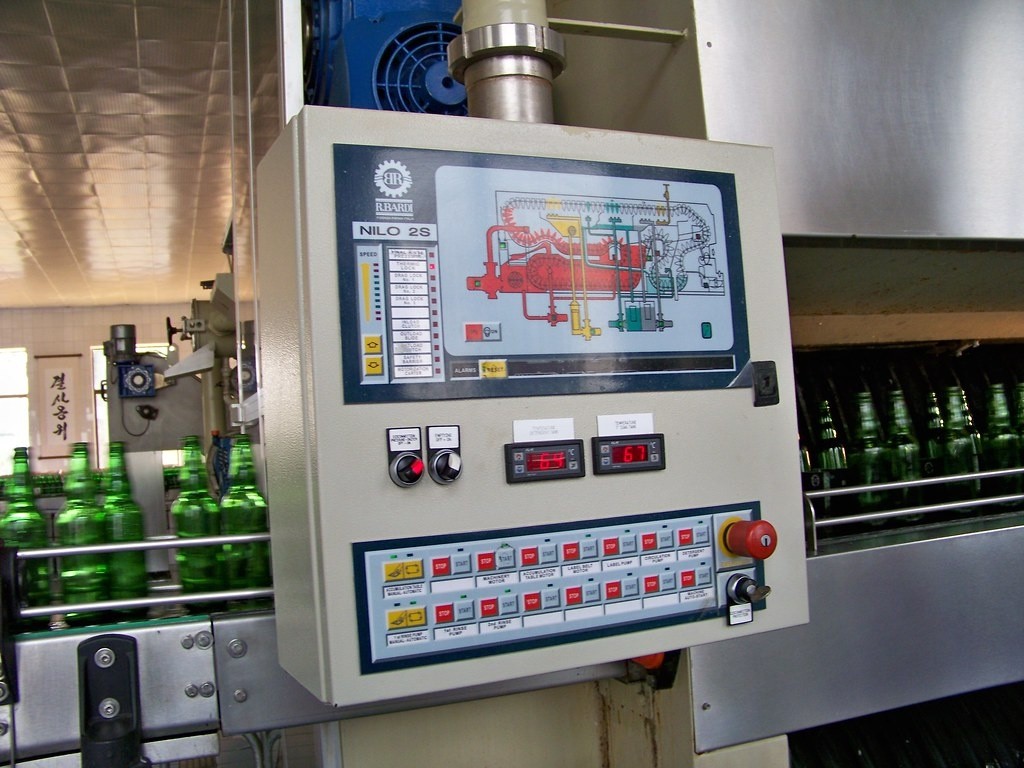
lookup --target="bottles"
[797,382,1024,524]
[0,436,269,620]
